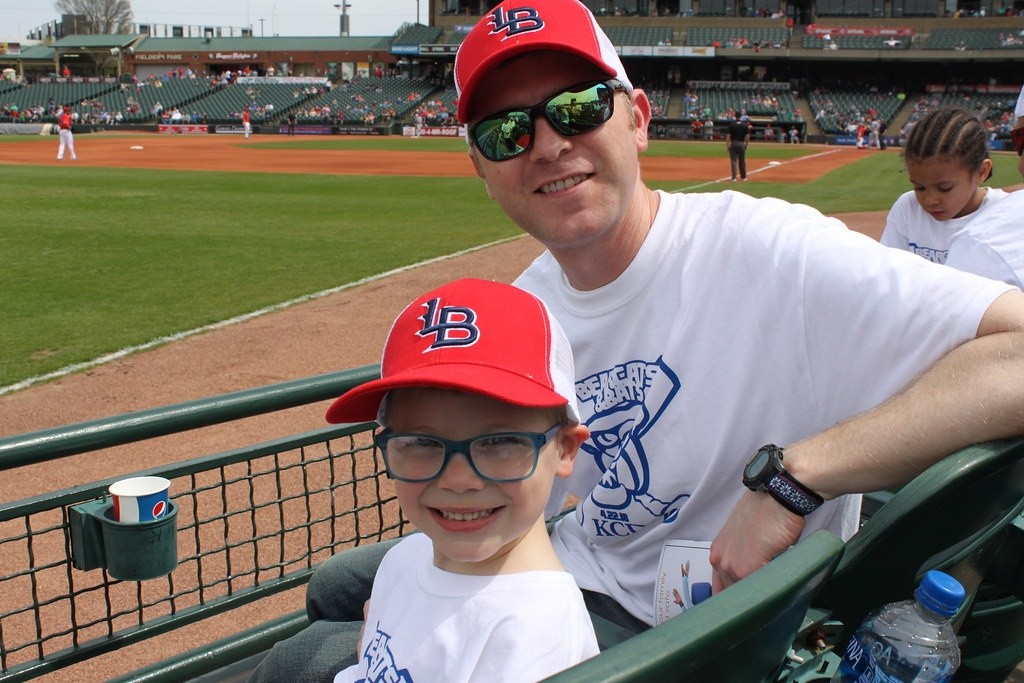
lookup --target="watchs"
[742,443,824,518]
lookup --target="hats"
[64,106,71,111]
[453,0,634,124]
[324,279,567,423]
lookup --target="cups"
[108,476,171,523]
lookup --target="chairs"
[542,439,1024,682]
[0,8,1023,183]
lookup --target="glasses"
[466,81,626,164]
[375,422,563,482]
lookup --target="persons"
[951,6,986,18]
[955,40,966,51]
[417,115,423,135]
[632,77,671,137]
[995,5,1024,17]
[287,109,297,136]
[818,31,838,50]
[242,106,251,138]
[0,64,466,128]
[707,8,794,52]
[554,87,611,126]
[500,114,530,156]
[726,111,750,182]
[998,28,1024,47]
[597,4,694,18]
[879,82,1024,292]
[683,70,888,151]
[658,38,671,46]
[56,107,77,160]
[324,278,600,683]
[882,36,901,47]
[241,0,1024,683]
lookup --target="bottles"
[829,570,966,683]
